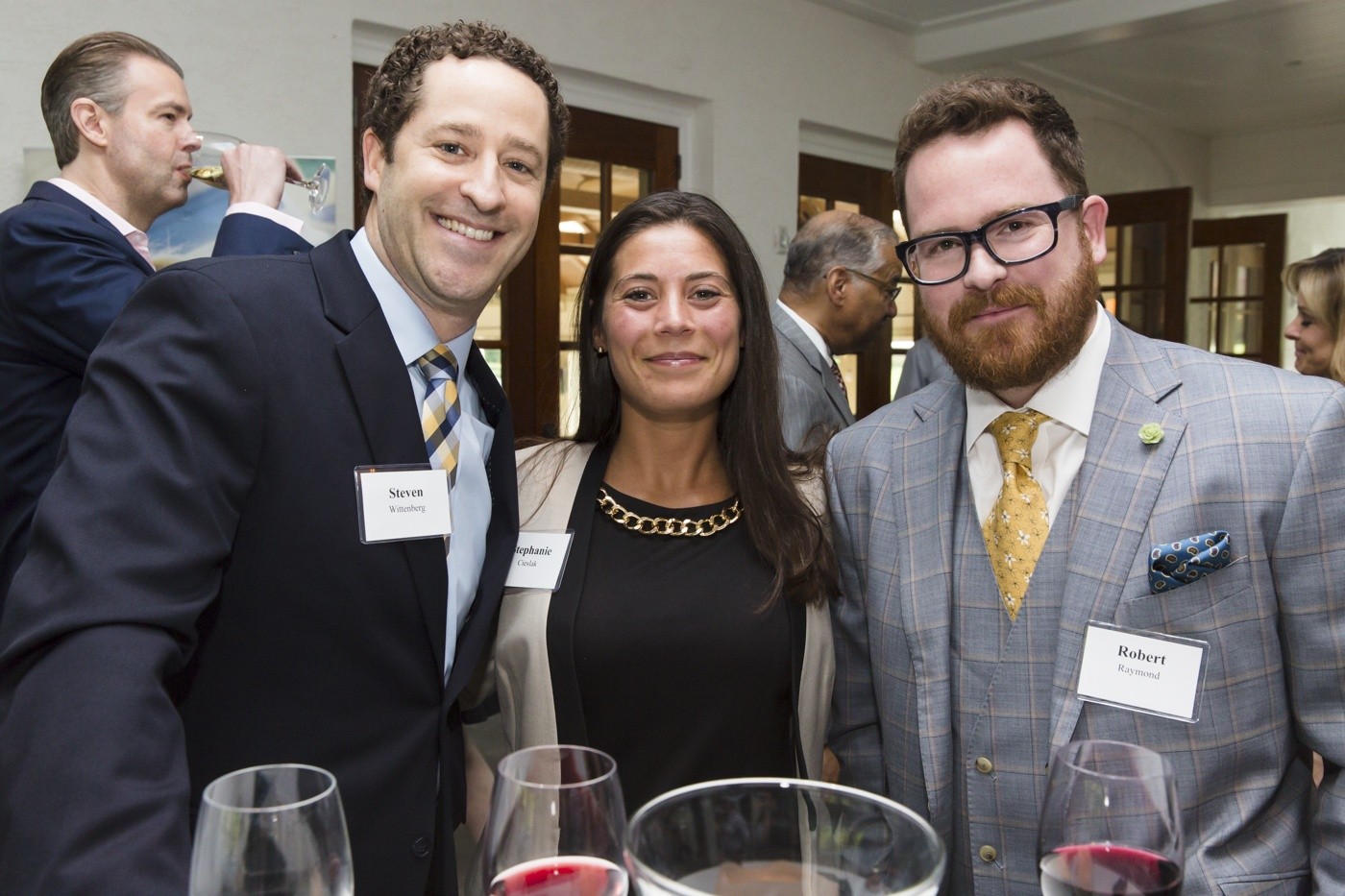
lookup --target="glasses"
[823,268,902,305]
[895,193,1082,285]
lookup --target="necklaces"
[597,485,744,539]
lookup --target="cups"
[622,778,947,895]
[482,744,630,895]
[1035,740,1184,895]
[187,763,356,895]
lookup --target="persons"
[816,76,1345,896]
[0,21,569,896]
[460,195,841,896]
[1284,248,1345,385]
[0,30,315,609]
[761,209,955,468]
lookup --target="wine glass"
[176,129,332,216]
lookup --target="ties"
[417,344,461,557]
[830,357,847,399]
[980,407,1052,623]
